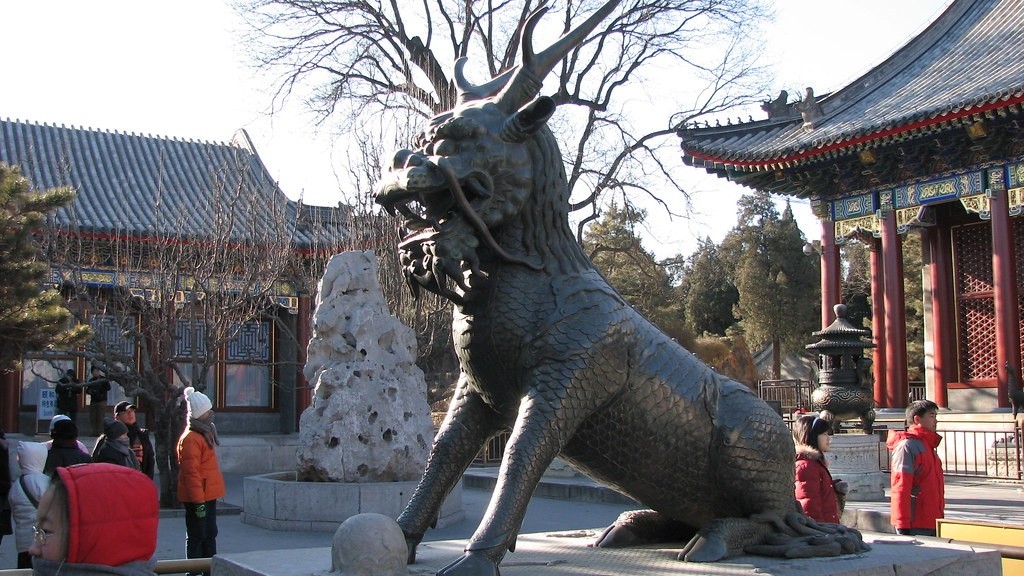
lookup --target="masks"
[122,438,130,446]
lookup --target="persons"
[0,364,159,576]
[886,399,945,538]
[795,401,808,416]
[176,387,226,576]
[790,417,840,524]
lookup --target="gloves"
[193,502,208,520]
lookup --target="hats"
[103,417,129,440]
[184,387,212,419]
[53,419,78,441]
[114,401,137,415]
[49,414,71,436]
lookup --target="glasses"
[32,525,68,544]
